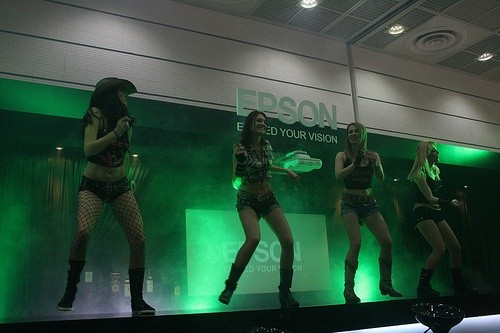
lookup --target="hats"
[89,78,137,105]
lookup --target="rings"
[235,149,237,151]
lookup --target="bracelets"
[376,166,380,170]
[438,199,458,208]
[114,129,120,137]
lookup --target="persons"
[408,140,479,296]
[58,76,155,312]
[334,121,403,303]
[218,111,300,307]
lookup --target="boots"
[417,268,439,297]
[344,260,360,303]
[128,268,156,314]
[451,267,476,294]
[57,260,87,311]
[278,268,299,308]
[219,264,245,305]
[378,257,402,297]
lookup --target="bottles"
[415,302,464,333]
